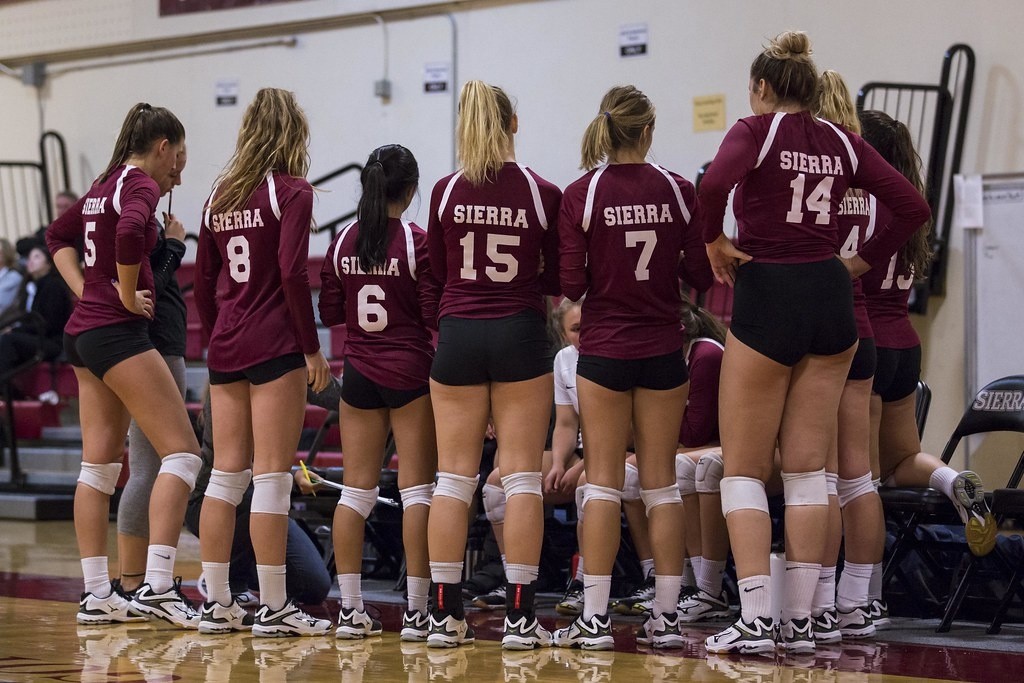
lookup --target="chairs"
[290,409,406,585]
[878,370,1024,634]
[734,379,931,619]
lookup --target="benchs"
[0,237,739,488]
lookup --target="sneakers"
[613,567,656,614]
[812,610,843,645]
[76,578,149,624]
[775,617,816,654]
[555,580,584,613]
[704,617,775,654]
[198,571,258,606]
[199,594,253,634]
[472,584,506,609]
[399,608,426,642]
[425,608,477,647]
[252,598,333,637]
[75,622,890,683]
[836,607,876,639]
[130,576,197,628]
[335,601,383,639]
[630,597,657,615]
[951,471,997,557]
[502,612,553,651]
[636,612,683,649]
[552,611,615,649]
[675,584,731,621]
[868,599,892,631]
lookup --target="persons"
[855,108,996,628]
[694,32,927,654]
[0,79,730,648]
[807,67,881,643]
